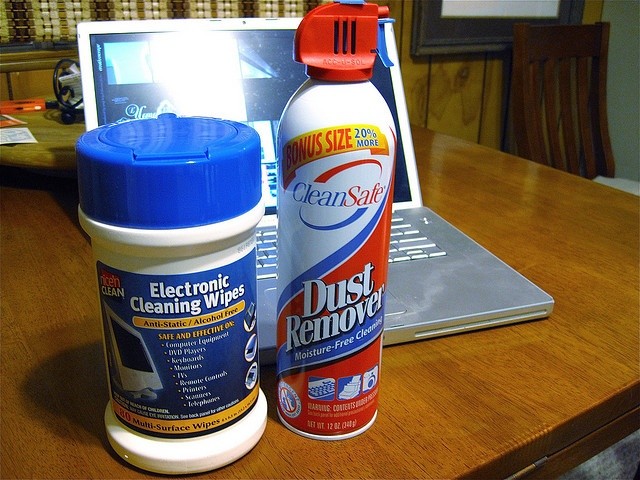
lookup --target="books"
[0,98,47,113]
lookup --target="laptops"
[76,17,556,370]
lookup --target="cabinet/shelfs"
[0,48,79,103]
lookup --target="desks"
[0,123,639,480]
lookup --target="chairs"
[511,22,615,178]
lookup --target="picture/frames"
[409,0,586,59]
